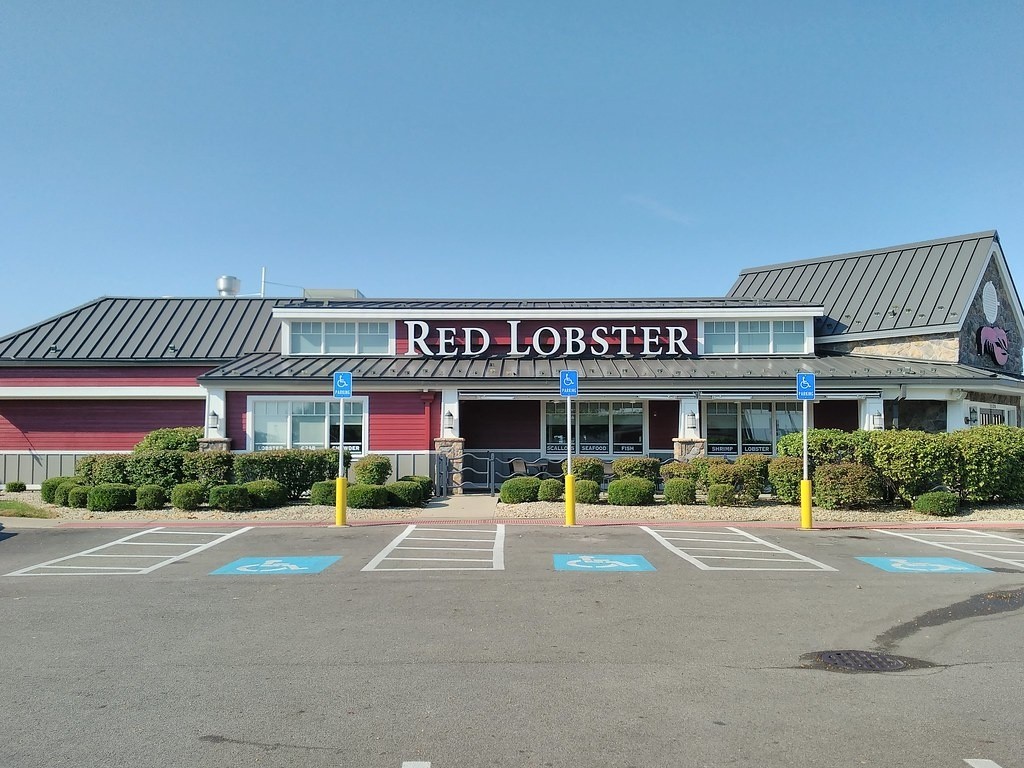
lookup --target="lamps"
[873,410,883,429]
[445,410,453,429]
[209,410,218,428]
[687,410,696,428]
[971,408,978,423]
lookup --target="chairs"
[508,457,514,478]
[511,459,527,477]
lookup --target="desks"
[525,464,548,478]
